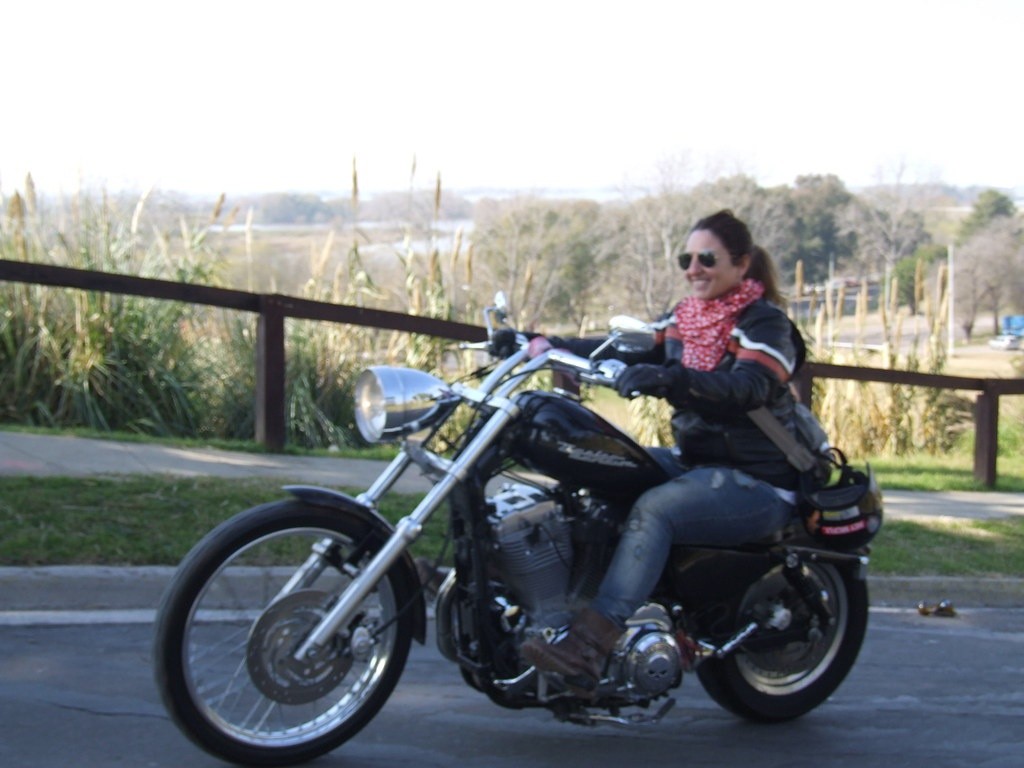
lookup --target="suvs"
[988,334,1019,349]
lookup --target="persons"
[491,210,808,696]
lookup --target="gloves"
[613,362,677,400]
[488,328,543,363]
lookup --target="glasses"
[678,251,739,269]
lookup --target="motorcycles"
[151,290,882,768]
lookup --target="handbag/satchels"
[798,464,883,548]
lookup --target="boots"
[518,609,623,696]
